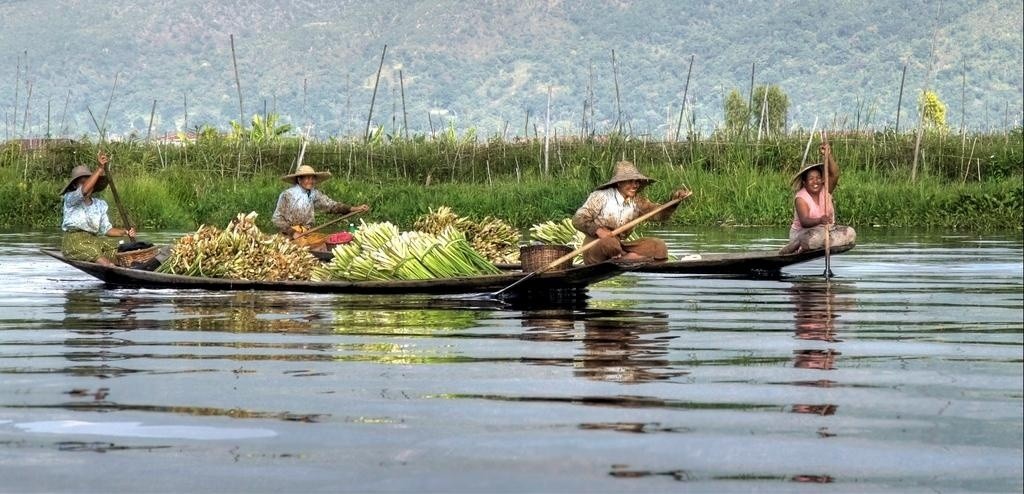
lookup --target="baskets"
[325,233,353,251]
[519,245,574,273]
[114,244,159,267]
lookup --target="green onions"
[153,206,678,282]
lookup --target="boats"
[48,243,655,292]
[629,237,857,276]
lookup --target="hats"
[790,164,825,190]
[596,161,658,191]
[280,165,331,186]
[59,165,109,196]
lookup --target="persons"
[572,160,686,265]
[58,151,136,267]
[270,164,366,252]
[776,140,855,254]
